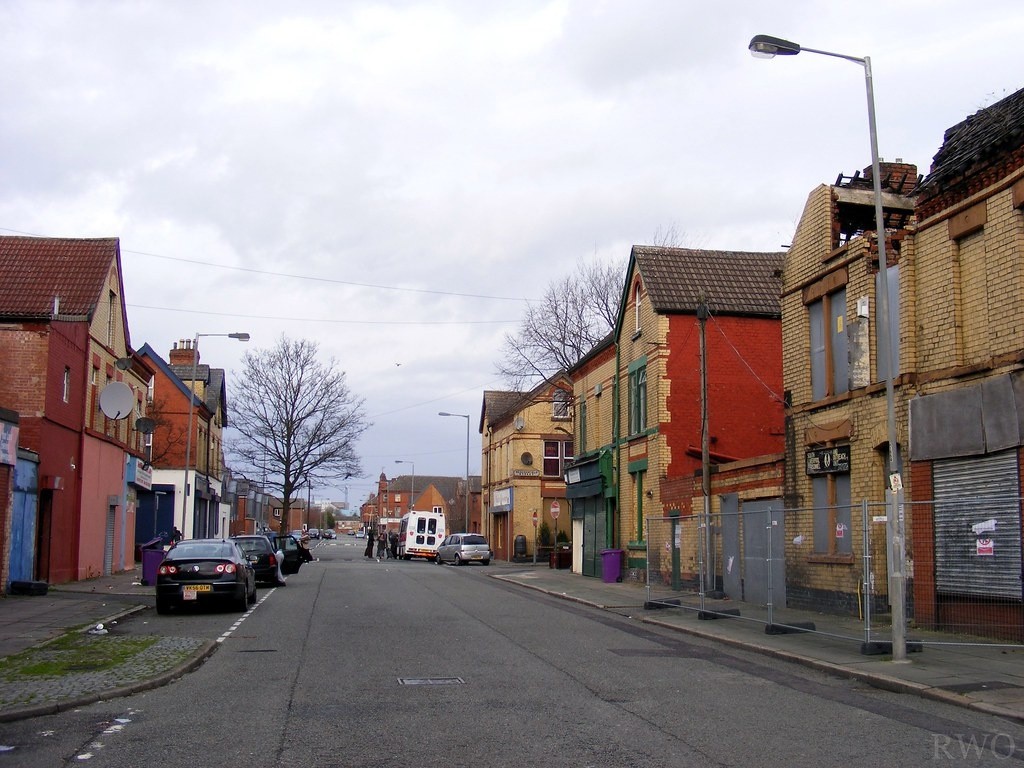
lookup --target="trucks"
[397,510,446,562]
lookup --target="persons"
[381,524,387,559]
[389,529,401,560]
[376,533,387,563]
[365,529,375,559]
[171,526,185,543]
[296,529,311,564]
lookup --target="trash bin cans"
[141,536,168,586]
[600,548,622,582]
[515,535,527,558]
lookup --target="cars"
[155,538,257,614]
[263,528,309,546]
[227,534,304,584]
[356,531,364,538]
[319,528,337,539]
[348,530,355,535]
[309,528,319,539]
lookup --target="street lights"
[349,481,389,532]
[439,411,470,533]
[395,460,414,511]
[751,32,910,664]
[181,332,250,543]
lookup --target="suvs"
[436,532,490,567]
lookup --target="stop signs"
[550,501,560,519]
[533,512,538,527]
